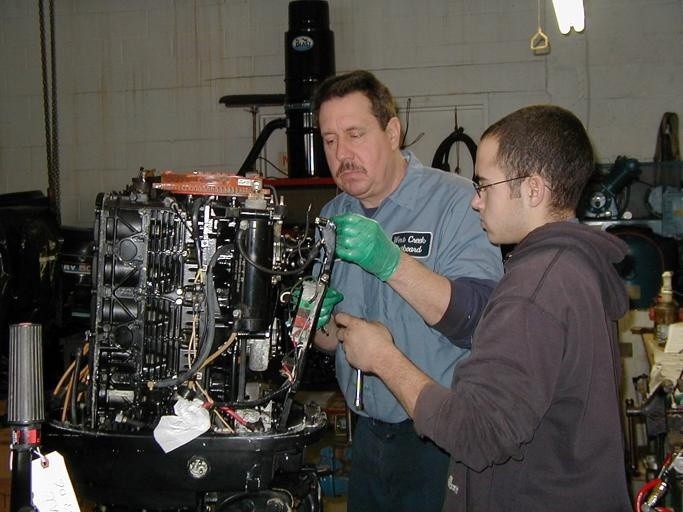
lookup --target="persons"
[334,104,633,511]
[296,72,505,512]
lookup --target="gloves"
[291,276,344,334]
[330,209,402,282]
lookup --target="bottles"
[655,271,677,348]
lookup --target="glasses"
[472,175,554,199]
